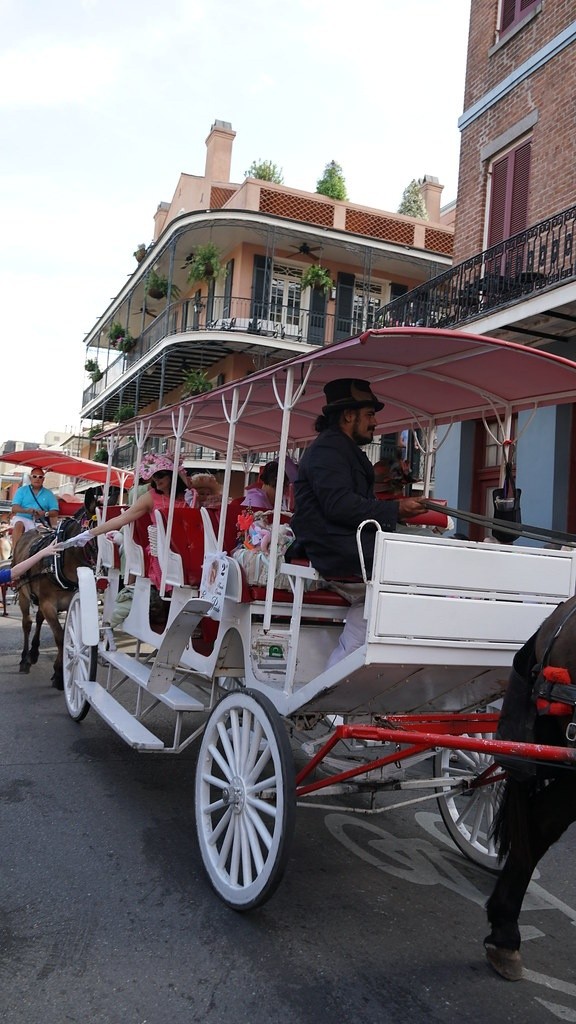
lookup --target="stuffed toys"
[236,513,270,551]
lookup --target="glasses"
[30,474,44,480]
[152,471,171,479]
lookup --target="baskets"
[229,510,328,592]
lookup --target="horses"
[481,595,576,982]
[8,486,117,691]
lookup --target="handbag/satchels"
[493,463,522,543]
[109,584,164,627]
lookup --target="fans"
[131,297,157,319]
[286,242,324,263]
[180,251,199,269]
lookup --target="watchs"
[44,511,48,517]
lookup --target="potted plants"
[105,322,138,352]
[141,267,182,303]
[112,401,136,424]
[180,368,213,396]
[297,267,337,300]
[186,242,233,282]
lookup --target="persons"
[0,523,12,541]
[11,467,59,549]
[117,469,153,580]
[0,538,64,585]
[191,473,232,506]
[66,450,198,594]
[240,456,299,510]
[290,378,429,671]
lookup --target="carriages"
[60,327,576,983]
[0,450,134,692]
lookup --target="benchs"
[101,496,447,623]
[9,501,85,536]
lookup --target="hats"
[139,450,192,489]
[184,474,221,507]
[276,455,299,483]
[322,378,384,415]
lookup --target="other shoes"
[191,626,202,639]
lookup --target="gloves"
[66,530,96,548]
